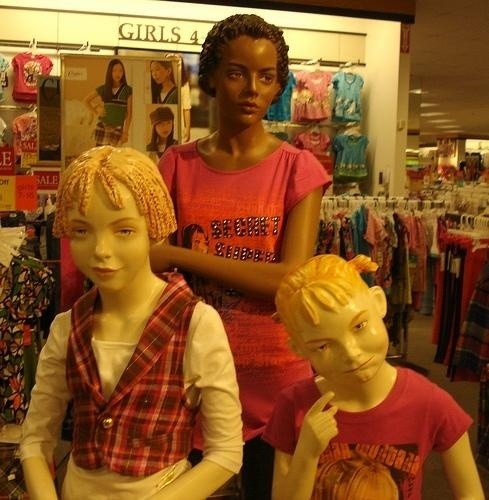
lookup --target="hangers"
[0,39,95,116]
[281,58,367,138]
[321,197,436,214]
[448,212,489,238]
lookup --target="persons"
[165,53,192,141]
[83,56,132,146]
[150,60,178,104]
[143,107,177,160]
[20,146,247,500]
[258,250,486,500]
[149,12,333,500]
